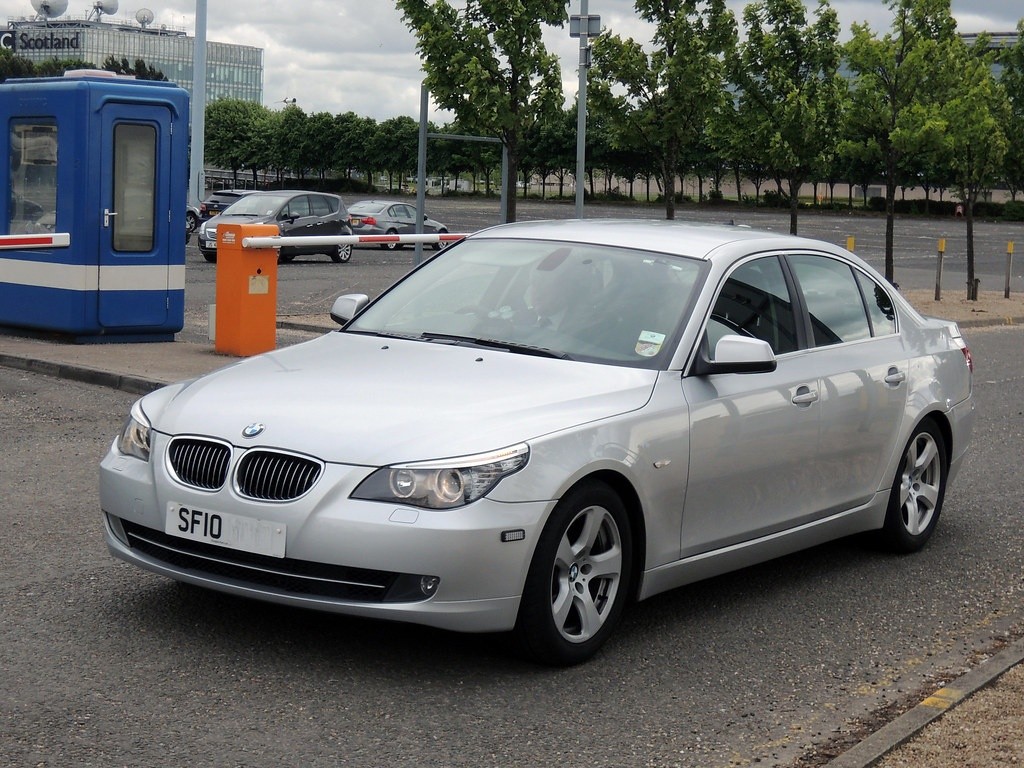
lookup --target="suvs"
[198,191,353,263]
[200,189,255,221]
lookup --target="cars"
[347,198,449,251]
[98,218,974,661]
[36,189,200,237]
[10,194,44,222]
